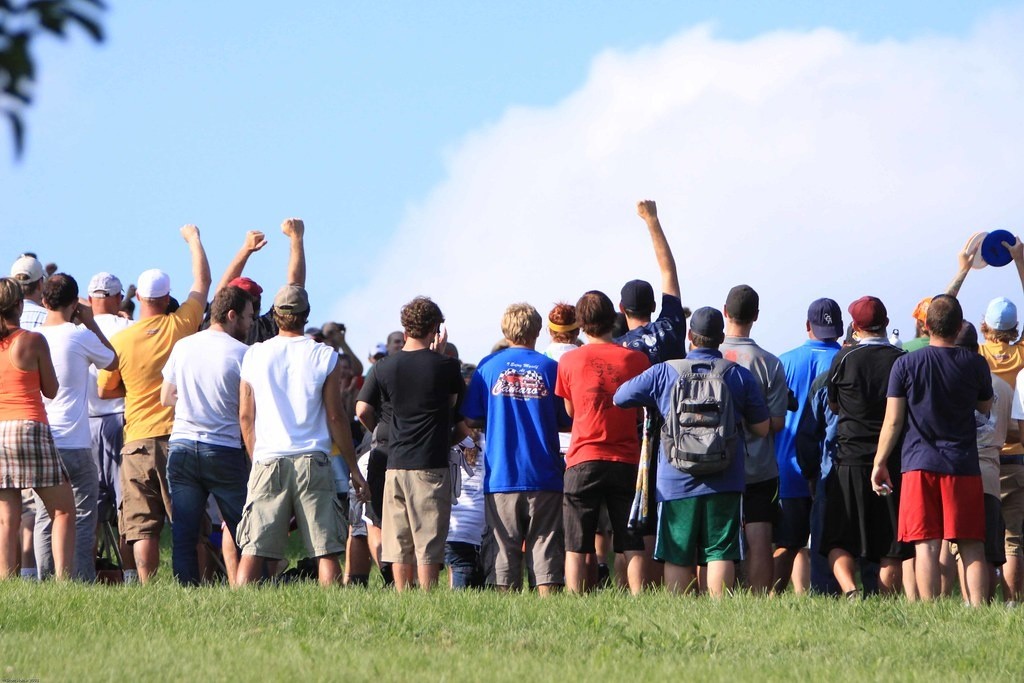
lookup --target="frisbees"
[982,229,1017,268]
[967,231,989,269]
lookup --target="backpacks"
[661,359,740,474]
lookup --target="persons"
[0,200,1024,605]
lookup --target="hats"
[848,296,887,332]
[274,285,308,314]
[369,343,387,357]
[10,255,50,283]
[985,298,1017,330]
[690,307,724,337]
[137,270,171,299]
[228,278,262,295]
[912,298,934,322]
[727,284,759,316]
[86,273,126,298]
[622,280,653,312]
[808,298,845,338]
